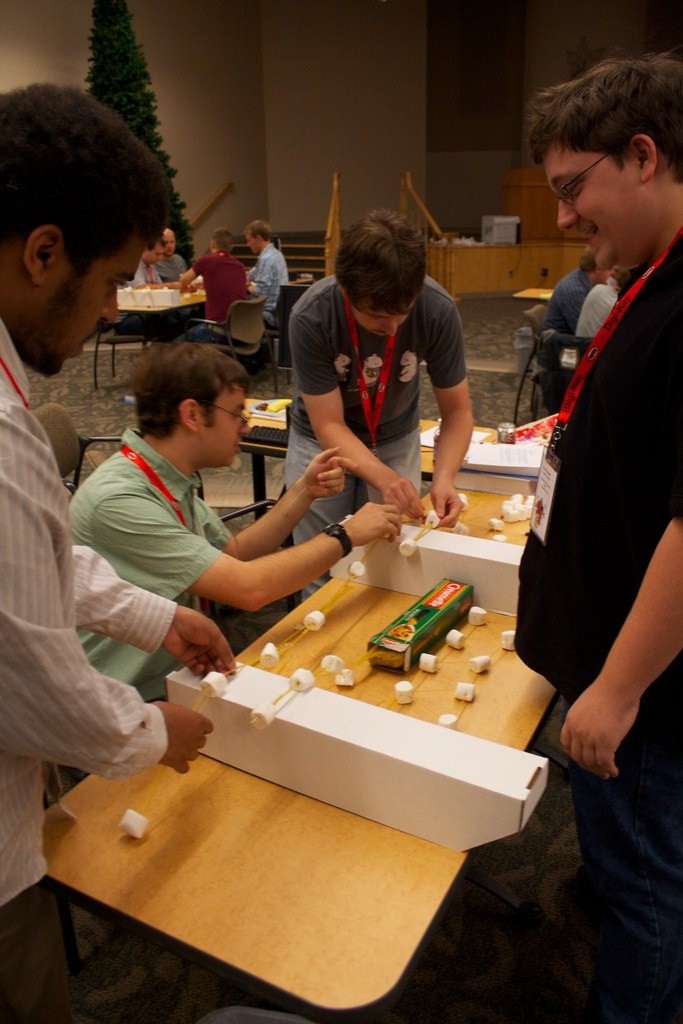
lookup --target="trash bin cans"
[513,327,534,375]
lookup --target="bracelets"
[325,522,353,558]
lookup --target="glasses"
[555,150,616,205]
[192,398,251,427]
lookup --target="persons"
[1,85,235,1024]
[116,239,171,290]
[155,227,187,284]
[541,247,612,336]
[518,56,683,1023]
[65,345,403,784]
[279,209,473,604]
[575,268,625,342]
[180,229,247,346]
[244,219,292,330]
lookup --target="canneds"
[497,420,517,445]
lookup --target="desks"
[41,233,600,1011]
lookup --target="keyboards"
[244,425,291,446]
